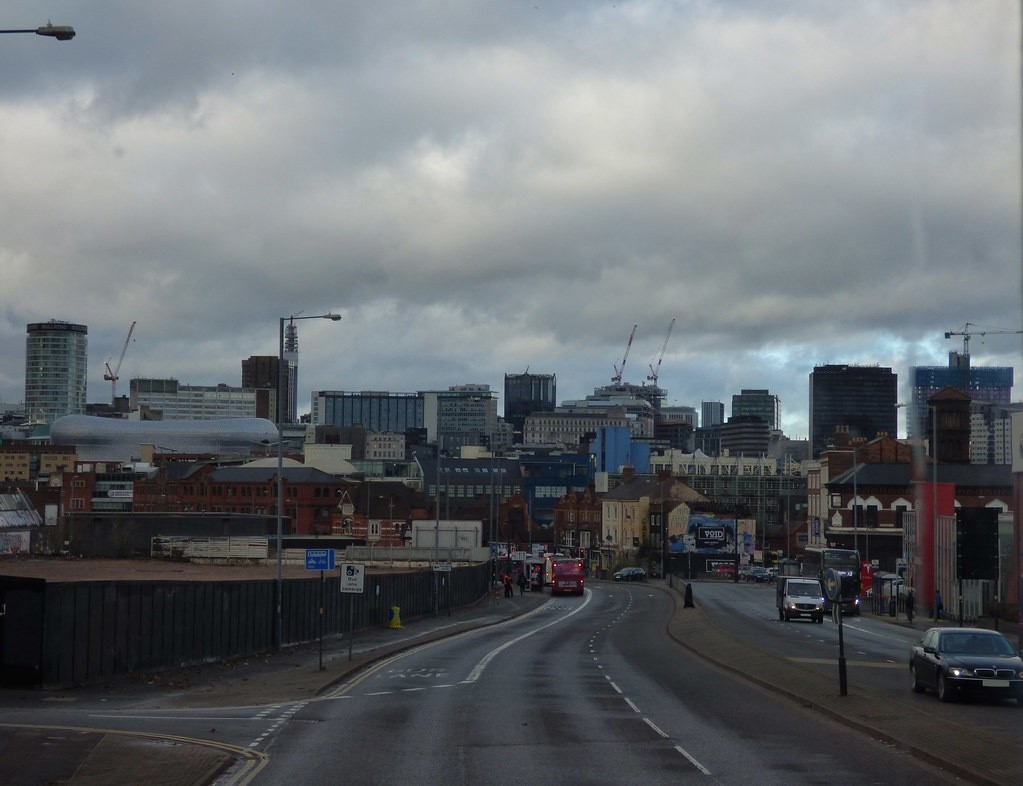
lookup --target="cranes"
[945,321,1023,367]
[102,321,136,403]
[611,325,636,386]
[642,318,677,388]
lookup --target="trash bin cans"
[889,600,896,617]
[388,605,401,629]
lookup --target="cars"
[614,567,648,581]
[732,547,801,584]
[910,626,1023,699]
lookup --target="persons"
[515,572,527,597]
[934,590,943,620]
[503,570,513,598]
[906,590,915,621]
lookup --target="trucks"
[774,575,824,623]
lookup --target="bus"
[545,553,564,584]
[805,548,861,616]
[550,560,583,596]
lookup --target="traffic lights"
[579,547,584,558]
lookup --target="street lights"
[272,312,341,654]
